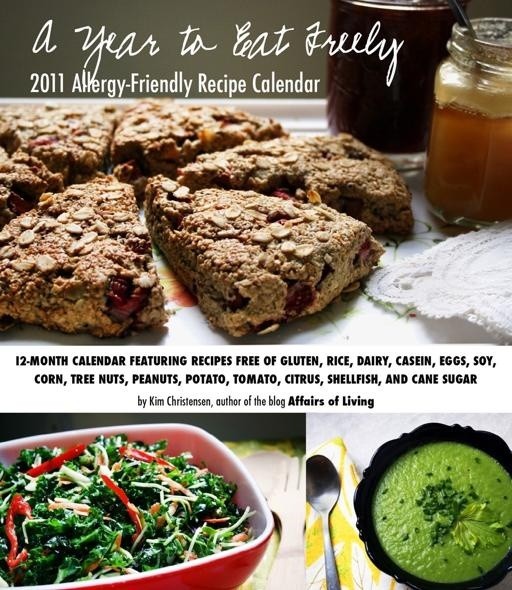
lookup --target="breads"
[0,99,414,339]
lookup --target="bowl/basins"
[1,423,273,590]
[354,421,511,590]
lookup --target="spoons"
[300,453,344,590]
